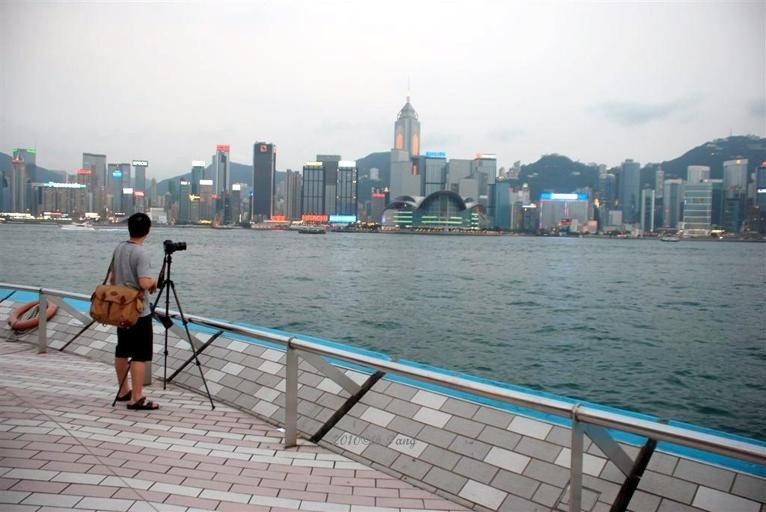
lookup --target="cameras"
[161,237,190,256]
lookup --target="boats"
[660,235,682,242]
[59,223,97,231]
[298,224,327,233]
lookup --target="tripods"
[112,258,216,417]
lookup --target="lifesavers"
[8,299,58,330]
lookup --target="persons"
[108,212,159,411]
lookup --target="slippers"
[116,389,159,410]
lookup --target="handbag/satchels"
[91,285,144,327]
